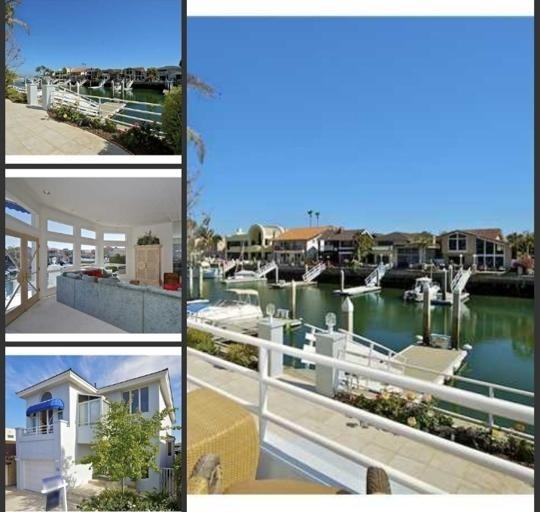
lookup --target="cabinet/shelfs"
[133,244,162,287]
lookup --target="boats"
[404,275,440,302]
[193,287,264,334]
[222,269,268,286]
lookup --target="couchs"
[55,268,182,334]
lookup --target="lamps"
[325,312,336,333]
[266,302,275,321]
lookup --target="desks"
[187,386,260,494]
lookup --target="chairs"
[186,451,393,495]
